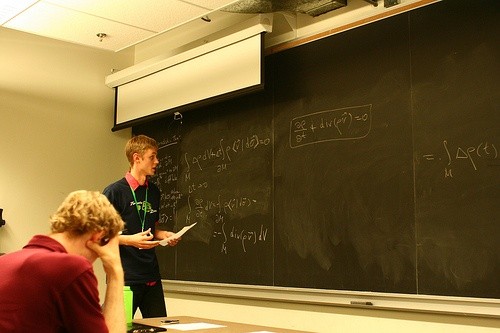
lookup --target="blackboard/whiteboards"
[131,0,500,302]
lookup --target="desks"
[127,315,306,332]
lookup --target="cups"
[124,285,133,330]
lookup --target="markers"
[350,301,374,306]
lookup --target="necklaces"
[131,187,148,232]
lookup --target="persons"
[0,190,127,333]
[101,135,181,320]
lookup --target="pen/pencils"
[161,319,180,322]
[161,322,179,325]
